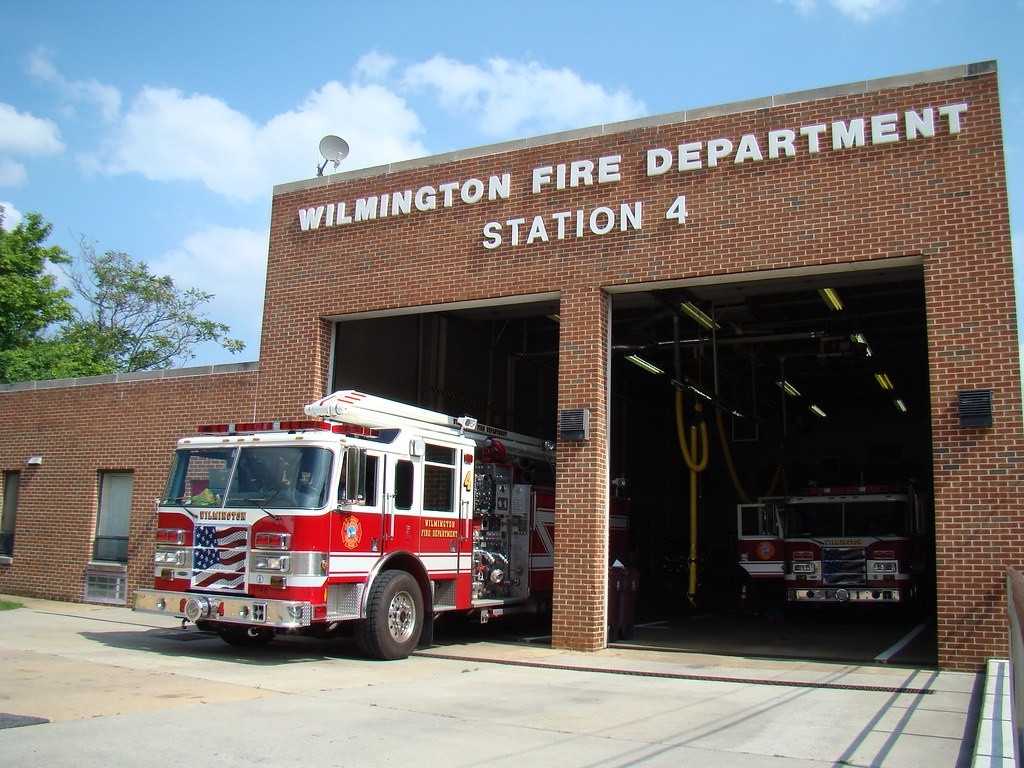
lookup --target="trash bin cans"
[606,566,635,643]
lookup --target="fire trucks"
[126,389,642,662]
[733,412,933,624]
